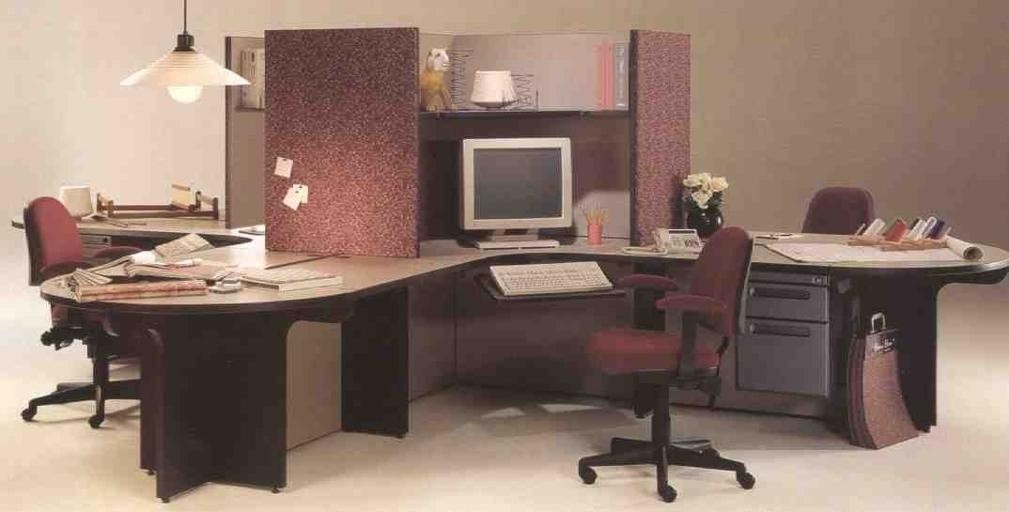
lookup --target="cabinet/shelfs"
[733,262,861,402]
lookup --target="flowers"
[682,170,729,210]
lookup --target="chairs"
[20,194,142,430]
[575,226,759,505]
[798,184,876,234]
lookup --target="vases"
[58,184,95,223]
[470,69,521,113]
[685,205,725,240]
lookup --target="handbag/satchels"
[844,311,921,447]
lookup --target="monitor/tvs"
[458,137,573,249]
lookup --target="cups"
[586,224,603,246]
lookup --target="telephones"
[652,227,703,253]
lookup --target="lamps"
[118,0,252,104]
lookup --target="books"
[47,231,342,305]
[598,41,630,106]
[763,238,984,264]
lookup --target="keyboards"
[488,261,613,297]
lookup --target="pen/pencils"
[585,208,605,225]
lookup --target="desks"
[9,203,1008,506]
